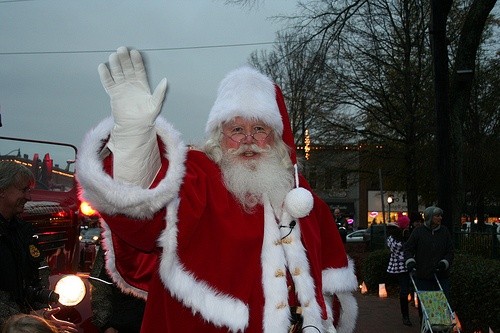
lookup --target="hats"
[206,69,313,218]
[397,212,410,229]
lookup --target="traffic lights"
[387,196,393,204]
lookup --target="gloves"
[432,262,446,274]
[407,262,416,273]
[98,46,168,190]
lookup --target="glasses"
[219,129,273,144]
[7,187,29,195]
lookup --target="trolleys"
[408,267,462,333]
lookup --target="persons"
[465,216,500,233]
[401,205,459,325]
[387,210,423,326]
[331,207,386,244]
[0,160,147,333]
[82,44,360,333]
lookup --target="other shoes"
[402,317,412,326]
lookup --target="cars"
[346,229,368,242]
[79,227,105,244]
